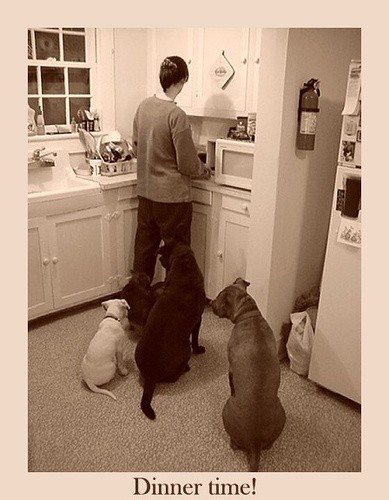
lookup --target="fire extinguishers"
[296,78,320,151]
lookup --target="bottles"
[236,116,248,140]
[70,116,76,133]
[37,106,45,135]
[247,112,257,137]
[94,115,99,131]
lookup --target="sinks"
[29,175,89,194]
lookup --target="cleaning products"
[36,105,46,136]
[27,104,37,136]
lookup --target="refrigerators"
[306,58,362,405]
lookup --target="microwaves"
[215,137,255,190]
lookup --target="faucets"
[33,146,58,167]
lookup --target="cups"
[90,159,101,177]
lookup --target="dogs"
[208,276,287,472]
[120,268,165,338]
[81,297,132,402]
[134,234,207,420]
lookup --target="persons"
[129,56,211,320]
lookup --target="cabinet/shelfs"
[149,28,194,116]
[28,189,110,320]
[115,184,163,292]
[215,188,250,304]
[184,189,212,298]
[207,28,261,118]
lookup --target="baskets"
[87,160,137,176]
